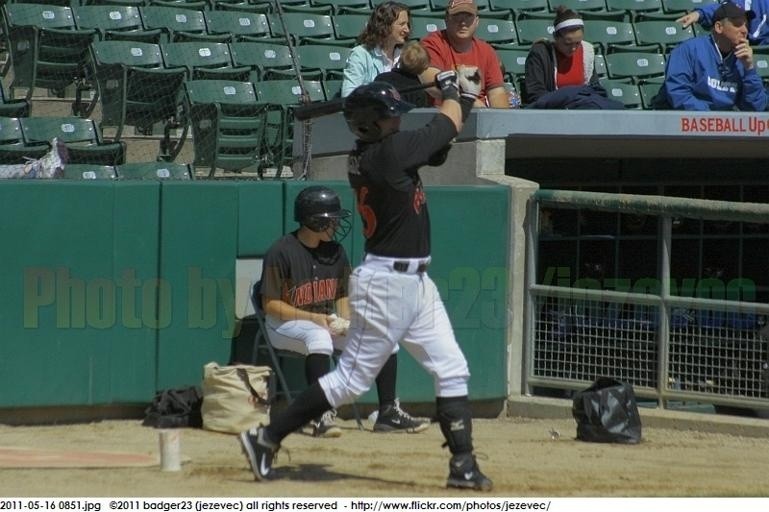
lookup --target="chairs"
[242,278,368,433]
[1,1,768,182]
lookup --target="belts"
[396,263,428,273]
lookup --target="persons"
[649,3,769,112]
[674,0,769,52]
[238,65,496,491]
[525,6,624,110]
[374,41,431,107]
[260,187,431,437]
[420,0,508,109]
[341,1,414,96]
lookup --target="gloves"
[436,70,459,101]
[458,64,481,101]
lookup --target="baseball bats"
[293,69,480,121]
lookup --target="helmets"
[343,81,416,137]
[294,186,353,245]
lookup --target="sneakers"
[239,423,280,482]
[36,137,69,178]
[447,456,493,490]
[374,397,430,433]
[310,408,341,437]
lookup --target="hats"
[712,2,756,23]
[447,0,477,16]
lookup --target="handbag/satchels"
[203,360,273,434]
[572,376,642,445]
[146,385,202,427]
